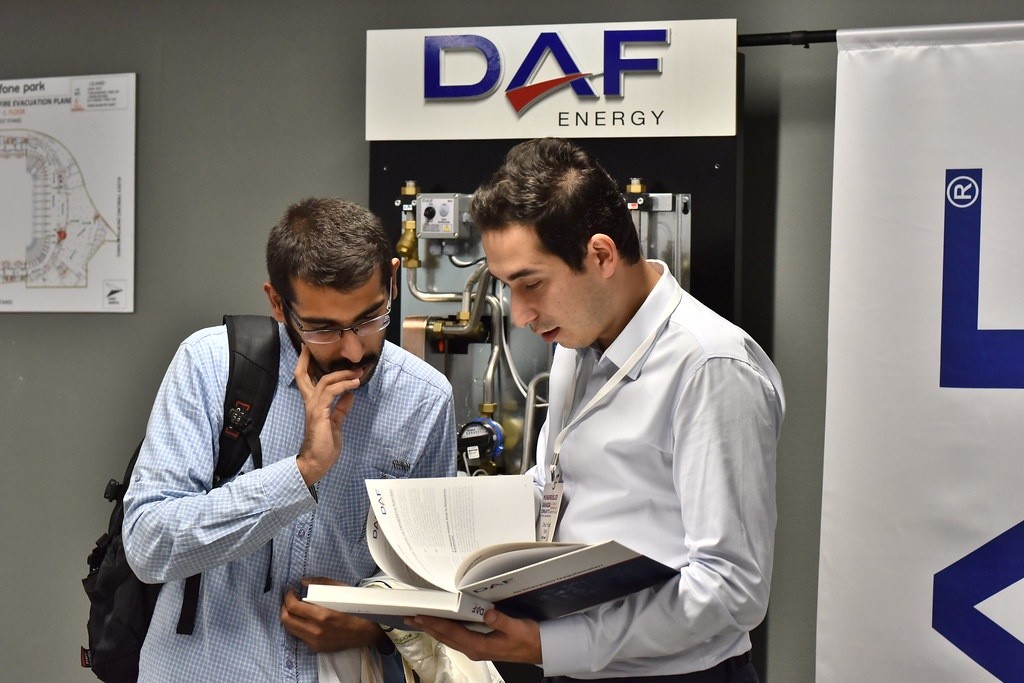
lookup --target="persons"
[403,137,786,683]
[122,195,457,683]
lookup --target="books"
[302,475,681,621]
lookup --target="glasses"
[283,277,393,346]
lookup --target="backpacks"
[79,313,280,683]
